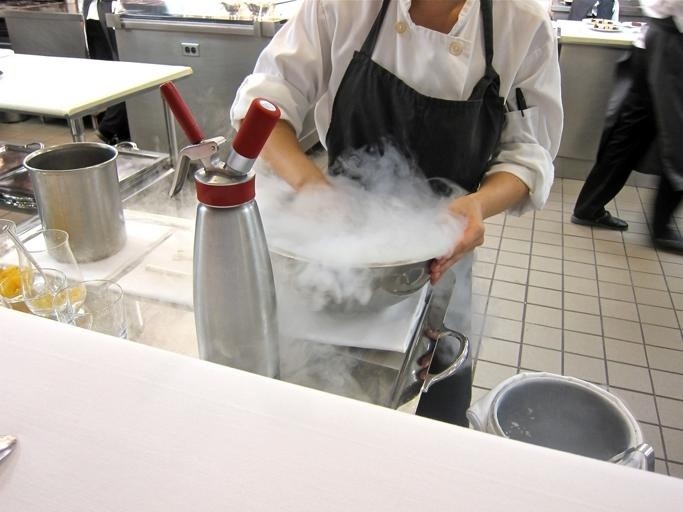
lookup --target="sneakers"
[93,129,119,145]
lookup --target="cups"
[22,141,127,263]
[49,280,128,338]
[0,219,31,303]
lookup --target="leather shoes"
[571,211,628,230]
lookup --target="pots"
[263,202,456,316]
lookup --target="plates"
[580,15,648,33]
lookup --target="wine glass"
[19,231,92,330]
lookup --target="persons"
[570,0,682,258]
[81,0,133,146]
[228,1,566,431]
